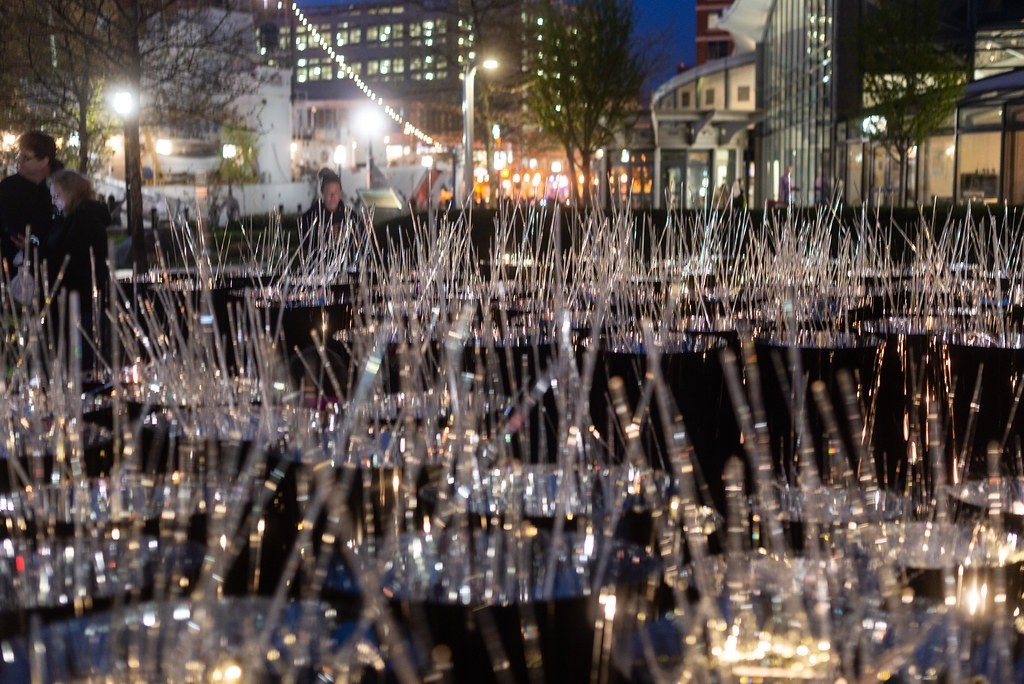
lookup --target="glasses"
[17,150,37,161]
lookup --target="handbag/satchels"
[7,267,37,306]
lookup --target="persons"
[732,178,746,210]
[208,199,220,230]
[0,128,111,371]
[815,166,828,203]
[99,192,126,228]
[779,166,796,201]
[221,192,239,227]
[299,172,364,245]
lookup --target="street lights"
[332,147,347,179]
[422,156,433,211]
[464,59,500,209]
[222,144,237,226]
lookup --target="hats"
[318,168,341,192]
